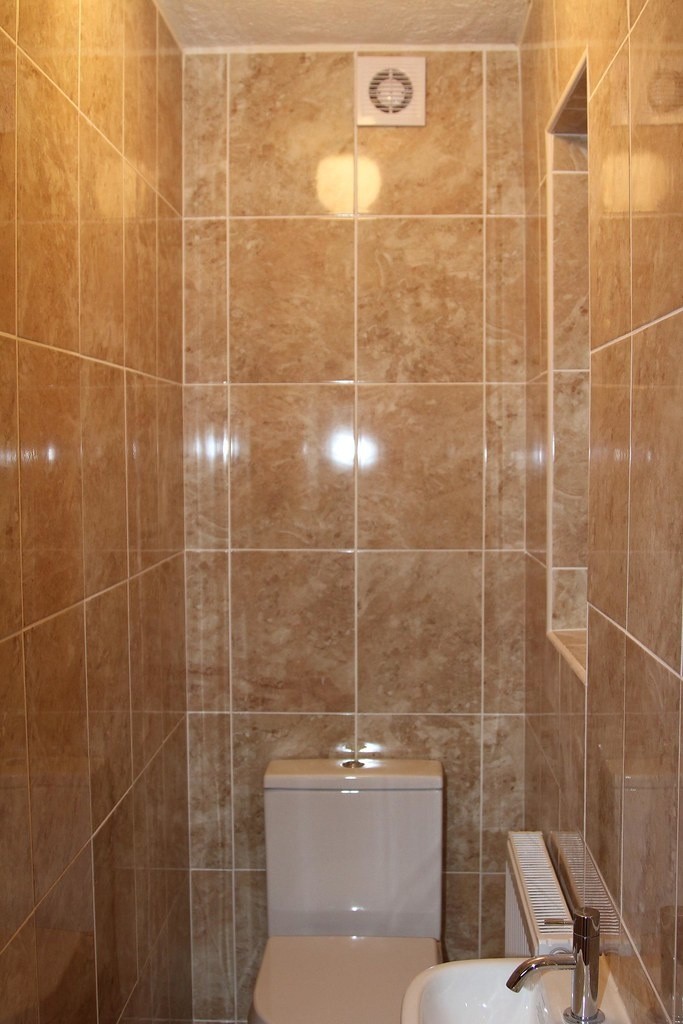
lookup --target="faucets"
[505,907,611,1024]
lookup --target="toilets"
[249,761,446,1023]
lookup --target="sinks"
[402,953,639,1023]
